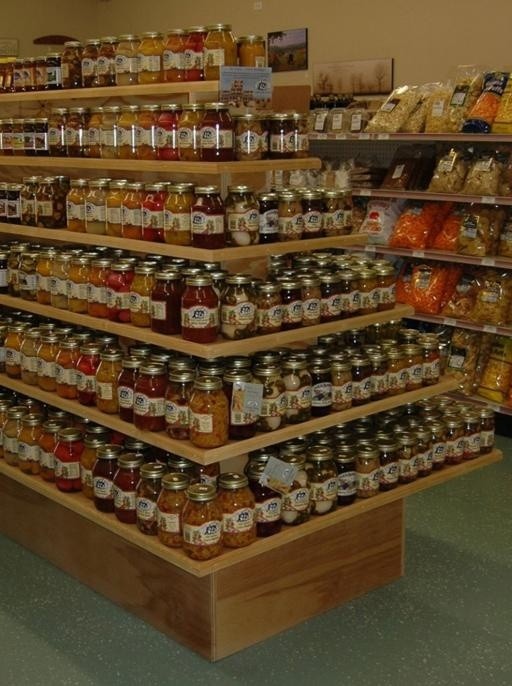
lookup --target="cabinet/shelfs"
[306,126,512,440]
[1,78,507,662]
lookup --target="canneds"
[1,239,396,344]
[0,175,354,249]
[1,311,441,448]
[2,102,310,162]
[1,387,495,561]
[1,24,266,94]
